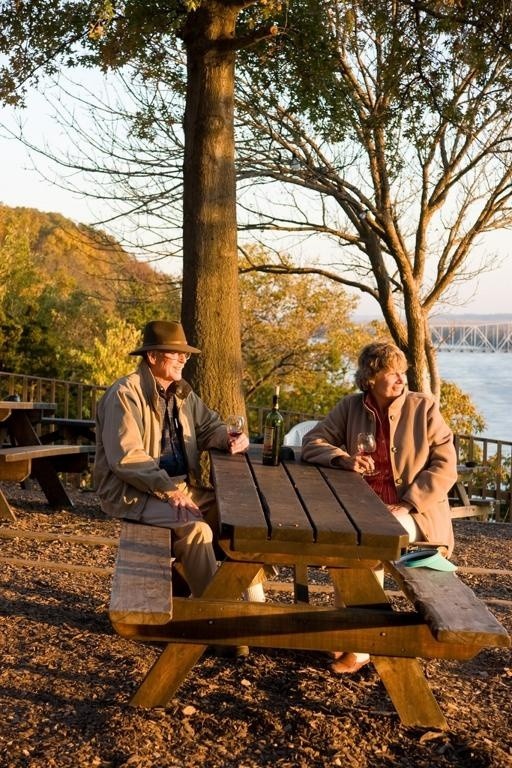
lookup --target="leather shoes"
[328,650,372,675]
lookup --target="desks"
[456,463,490,522]
[0,401,73,521]
[130,443,452,731]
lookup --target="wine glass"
[357,431,380,477]
[223,415,245,458]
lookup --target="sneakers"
[211,644,250,657]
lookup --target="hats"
[127,321,201,355]
[397,551,458,573]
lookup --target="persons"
[92,320,268,658]
[300,340,459,677]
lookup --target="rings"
[360,466,363,469]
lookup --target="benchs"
[373,555,510,730]
[107,516,208,718]
[0,444,96,522]
[448,496,497,523]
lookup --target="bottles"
[262,384,284,466]
[14,392,20,402]
